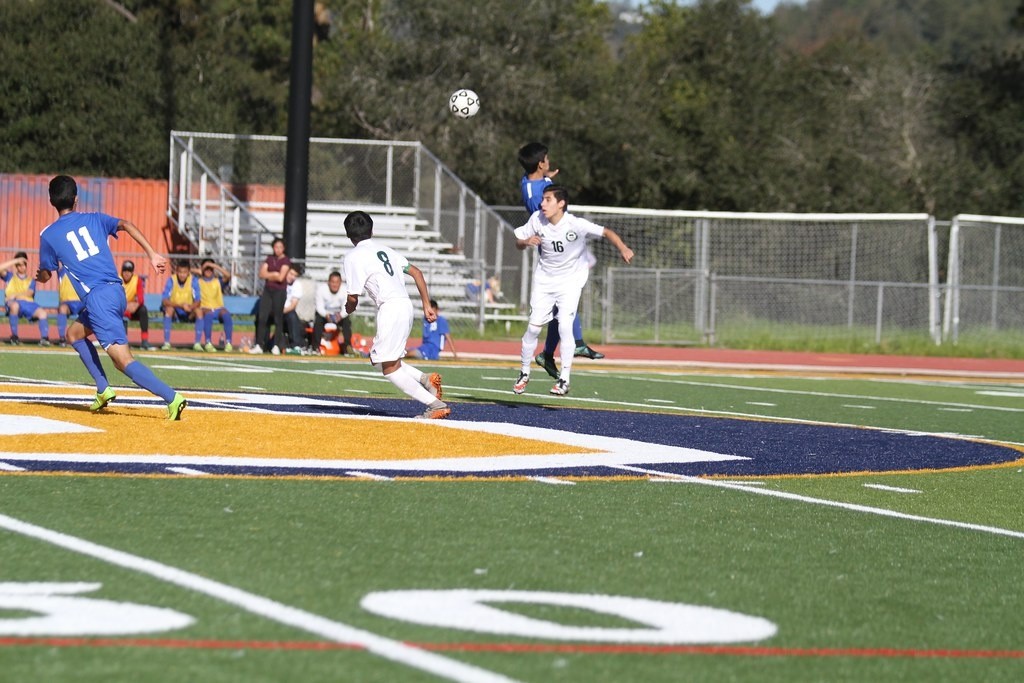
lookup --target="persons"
[55,260,86,347]
[517,141,605,380]
[466,273,499,304]
[333,210,452,418]
[118,260,158,351]
[0,252,54,347]
[248,238,290,355]
[34,174,188,421]
[308,271,354,358]
[406,300,456,359]
[255,265,304,354]
[190,259,233,352]
[159,263,204,352]
[512,184,634,396]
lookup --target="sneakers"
[59,340,66,348]
[574,344,604,359]
[40,338,51,347]
[11,335,19,346]
[425,373,443,400]
[249,343,263,354]
[193,342,204,352]
[549,379,570,395]
[271,346,280,356]
[162,342,171,351]
[89,386,116,411]
[535,353,561,379]
[512,371,530,394]
[225,342,232,353]
[413,404,451,419]
[140,341,157,351]
[204,342,217,353]
[167,392,188,420]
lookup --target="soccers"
[448,89,481,120]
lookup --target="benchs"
[0,198,530,349]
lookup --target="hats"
[122,260,134,271]
